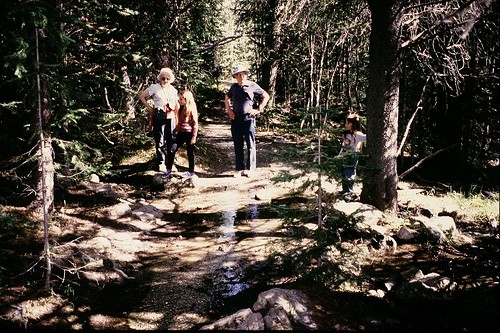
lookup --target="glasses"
[160,76,170,81]
[179,98,185,101]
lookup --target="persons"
[162,91,198,179]
[337,114,366,196]
[225,66,270,176]
[139,68,180,162]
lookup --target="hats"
[232,66,252,79]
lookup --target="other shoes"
[351,193,359,198]
[180,172,195,177]
[344,194,351,200]
[245,170,256,177]
[157,162,167,172]
[234,170,243,178]
[171,164,178,172]
[161,170,173,178]
